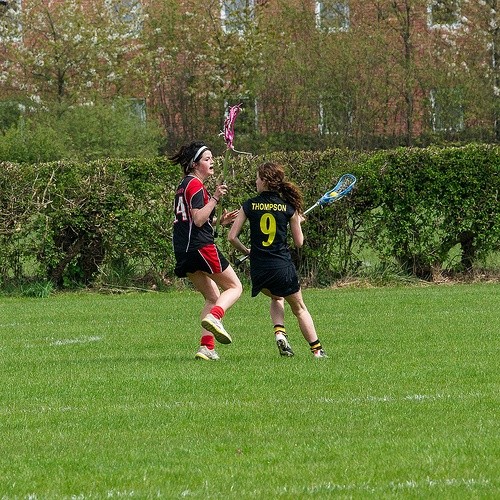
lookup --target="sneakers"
[275,332,294,357]
[313,349,329,358]
[201,314,232,344]
[195,346,220,361]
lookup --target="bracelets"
[212,196,218,202]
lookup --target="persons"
[167,142,239,361]
[227,162,330,359]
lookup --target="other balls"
[330,192,339,197]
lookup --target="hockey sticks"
[234,173,356,267]
[213,103,252,239]
